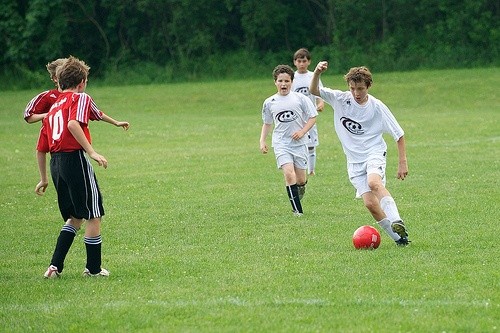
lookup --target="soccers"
[351,224,382,251]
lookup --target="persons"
[23,59,130,130]
[309,61,408,246]
[35,55,109,278]
[260,65,319,218]
[288,48,324,176]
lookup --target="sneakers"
[297,186,305,200]
[293,213,304,218]
[395,238,411,247]
[82,266,109,277]
[44,264,62,279]
[391,220,408,240]
